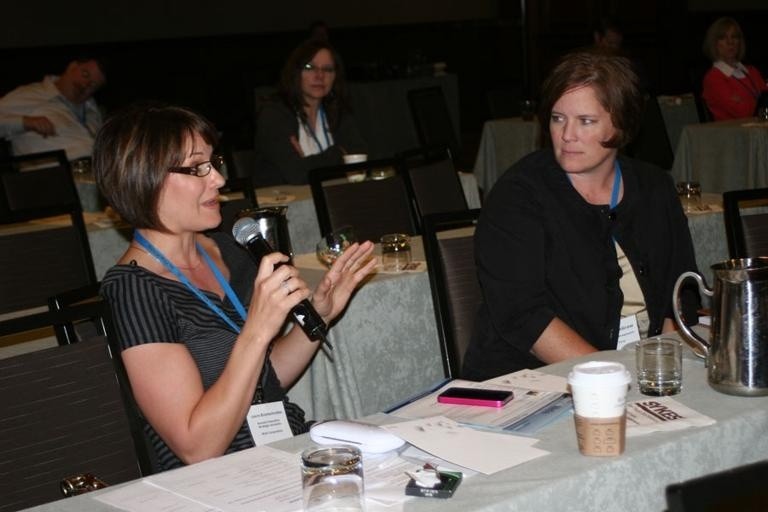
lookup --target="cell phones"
[437,387,514,408]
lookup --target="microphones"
[232,217,334,350]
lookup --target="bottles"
[216,153,230,181]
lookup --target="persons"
[252,40,366,186]
[461,50,699,382]
[702,16,768,121]
[310,21,329,42]
[0,55,106,212]
[92,101,377,471]
[589,17,625,53]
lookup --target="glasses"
[305,63,334,73]
[167,155,224,177]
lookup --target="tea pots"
[670,257,767,397]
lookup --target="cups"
[633,337,685,397]
[298,442,366,512]
[567,359,633,417]
[341,153,369,183]
[675,179,705,214]
[379,232,413,273]
